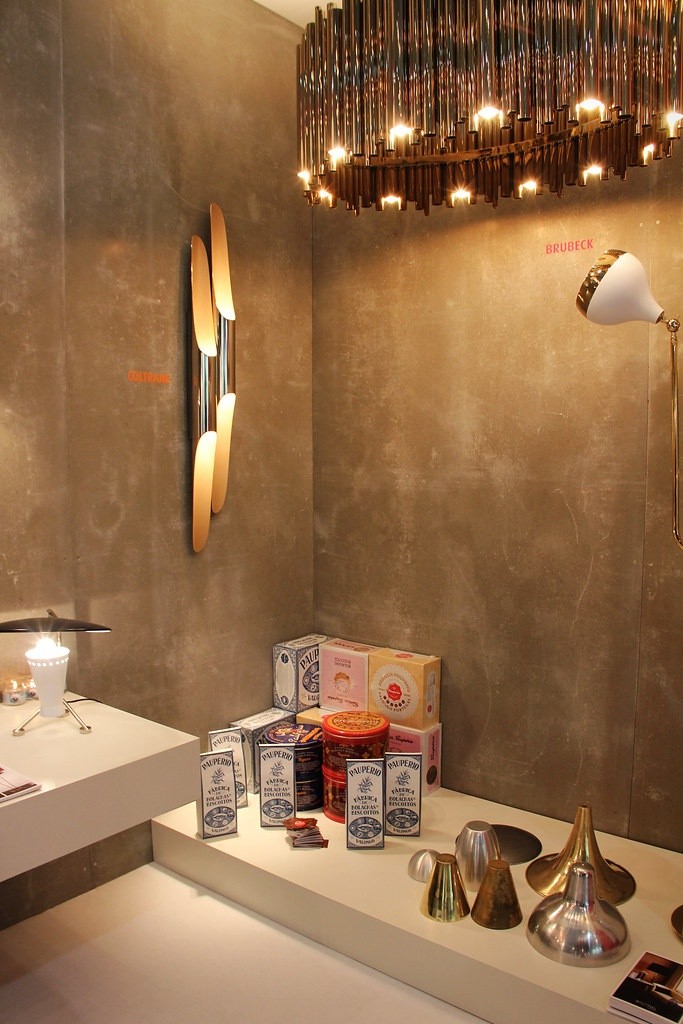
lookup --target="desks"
[0,691,200,882]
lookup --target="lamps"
[296,0,683,216]
[0,609,112,736]
[191,203,236,553]
[575,249,683,550]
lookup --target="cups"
[455,820,501,892]
[408,849,440,882]
[471,859,523,930]
[420,853,470,923]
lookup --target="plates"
[671,905,683,940]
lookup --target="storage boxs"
[229,633,442,797]
